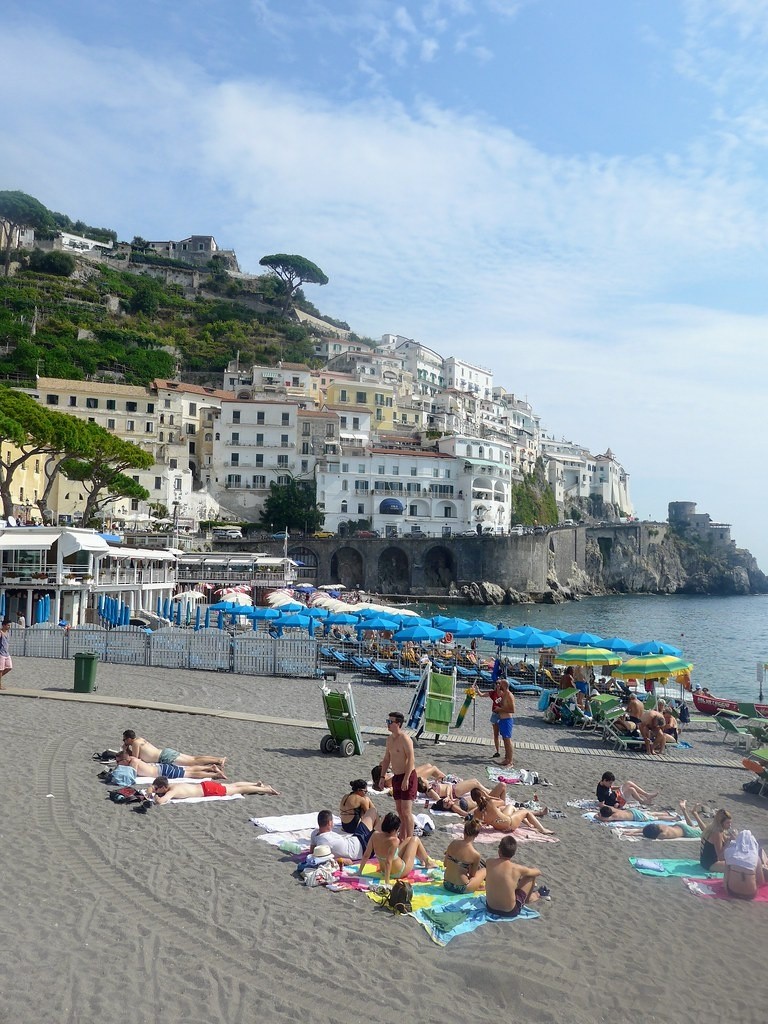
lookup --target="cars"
[482,518,609,537]
[312,531,334,539]
[355,531,376,539]
[270,531,291,540]
[403,530,428,538]
[460,529,479,537]
[212,529,243,540]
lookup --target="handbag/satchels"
[92,750,118,761]
[110,787,140,803]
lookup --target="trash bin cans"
[73,652,100,693]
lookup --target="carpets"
[108,749,768,947]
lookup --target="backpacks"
[380,879,413,915]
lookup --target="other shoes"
[143,801,154,808]
[134,805,147,814]
[493,752,500,757]
[514,802,529,808]
[533,885,551,901]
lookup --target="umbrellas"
[95,514,173,531]
[171,583,694,711]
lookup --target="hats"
[695,684,701,688]
[310,845,334,860]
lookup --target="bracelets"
[380,776,385,778]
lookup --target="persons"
[112,751,228,780]
[340,779,377,833]
[347,812,439,885]
[444,820,486,894]
[624,800,706,840]
[147,776,280,806]
[17,612,25,628]
[0,620,13,690]
[310,808,380,861]
[485,836,541,917]
[722,830,768,900]
[700,809,739,872]
[16,515,154,536]
[122,729,227,768]
[379,712,418,844]
[305,592,726,835]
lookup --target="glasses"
[359,789,368,794]
[155,786,160,790]
[495,681,500,685]
[122,737,130,742]
[117,759,126,763]
[386,719,399,724]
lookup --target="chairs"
[167,592,768,802]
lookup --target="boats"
[691,692,768,718]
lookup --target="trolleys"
[318,683,365,758]
